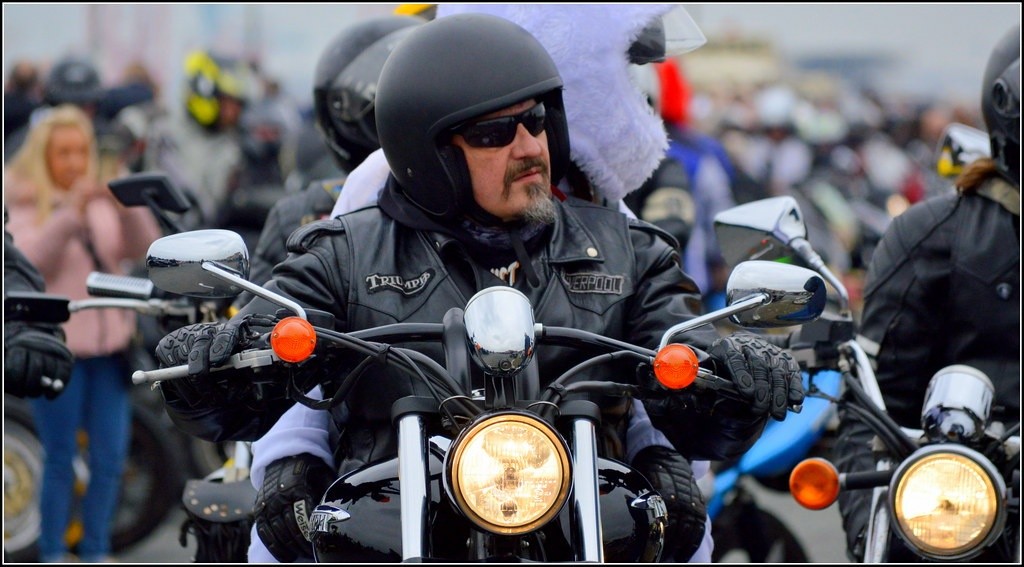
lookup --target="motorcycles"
[4,173,1019,564]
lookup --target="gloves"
[253,458,320,562]
[701,334,806,452]
[4,332,72,402]
[624,446,708,563]
[155,321,279,412]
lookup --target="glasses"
[453,101,546,148]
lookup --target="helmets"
[981,23,1021,187]
[47,63,100,105]
[314,17,426,175]
[184,51,264,125]
[374,12,571,215]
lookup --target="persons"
[157,13,805,563]
[3,2,1020,563]
